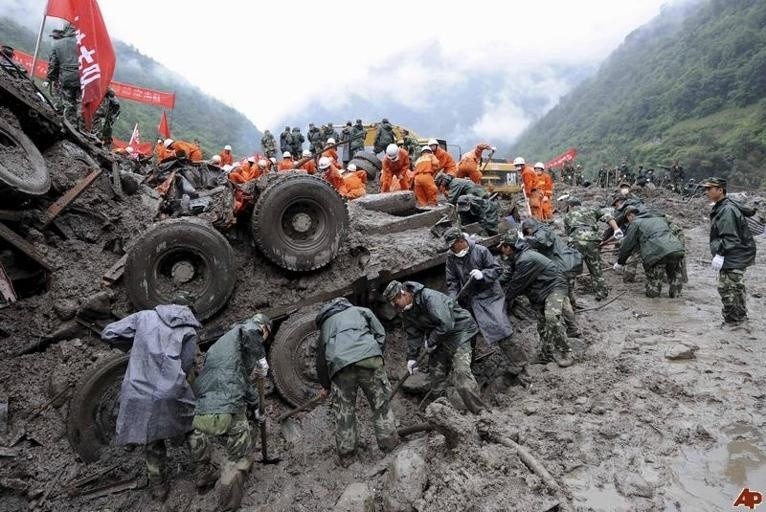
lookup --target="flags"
[43,0,117,131]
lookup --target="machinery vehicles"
[319,122,524,197]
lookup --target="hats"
[611,196,627,205]
[265,119,409,136]
[567,197,581,212]
[382,280,402,302]
[496,234,517,249]
[700,177,727,190]
[522,219,538,232]
[441,227,463,250]
[171,290,198,314]
[251,313,273,335]
[434,172,446,187]
[558,350,573,366]
[619,182,631,188]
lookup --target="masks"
[454,250,468,259]
[390,157,398,163]
[620,188,629,196]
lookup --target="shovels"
[254,366,282,464]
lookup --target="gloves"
[469,269,483,280]
[254,357,269,380]
[613,262,623,275]
[407,360,418,375]
[614,228,624,240]
[425,341,436,354]
[255,408,268,425]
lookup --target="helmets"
[386,143,399,159]
[428,138,439,146]
[326,138,336,144]
[158,138,292,175]
[346,164,356,172]
[513,157,525,166]
[303,150,310,157]
[420,146,432,154]
[534,162,545,171]
[319,157,331,170]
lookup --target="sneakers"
[195,463,220,488]
[152,480,169,501]
